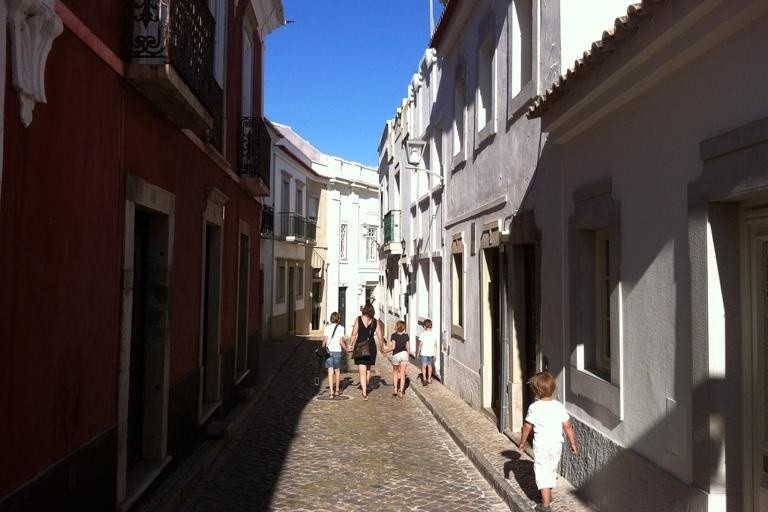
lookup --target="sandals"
[366,384,371,390]
[335,390,343,396]
[361,393,369,400]
[329,394,335,399]
[398,391,403,398]
[392,390,398,396]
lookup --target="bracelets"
[349,341,353,346]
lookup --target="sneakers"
[427,377,432,383]
[536,502,550,512]
[422,378,427,386]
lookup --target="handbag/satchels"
[322,347,329,357]
[352,338,370,359]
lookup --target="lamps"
[402,137,443,188]
[401,237,406,250]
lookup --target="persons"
[517,371,579,511]
[415,318,437,386]
[321,311,353,401]
[379,320,411,397]
[348,303,384,401]
